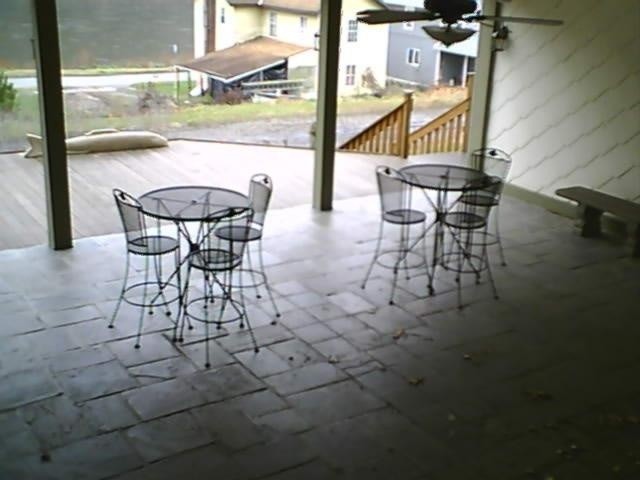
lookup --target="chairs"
[214,173,281,319]
[439,176,501,307]
[454,148,512,267]
[362,166,433,303]
[109,189,192,349]
[180,206,259,367]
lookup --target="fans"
[356,0,564,27]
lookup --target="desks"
[136,185,252,330]
[399,165,489,292]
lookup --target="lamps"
[422,1,475,46]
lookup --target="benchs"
[556,187,639,254]
